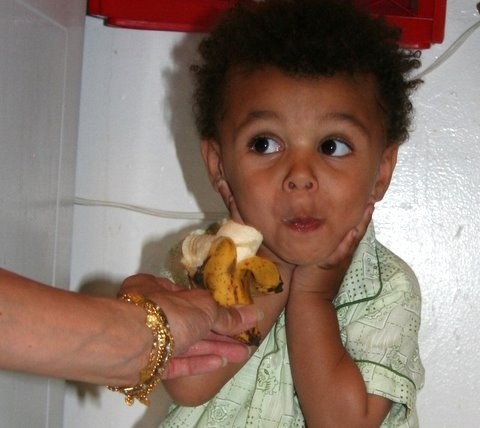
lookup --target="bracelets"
[104,290,175,407]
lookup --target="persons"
[1,266,266,390]
[156,3,423,428]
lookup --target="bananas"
[179,219,284,348]
